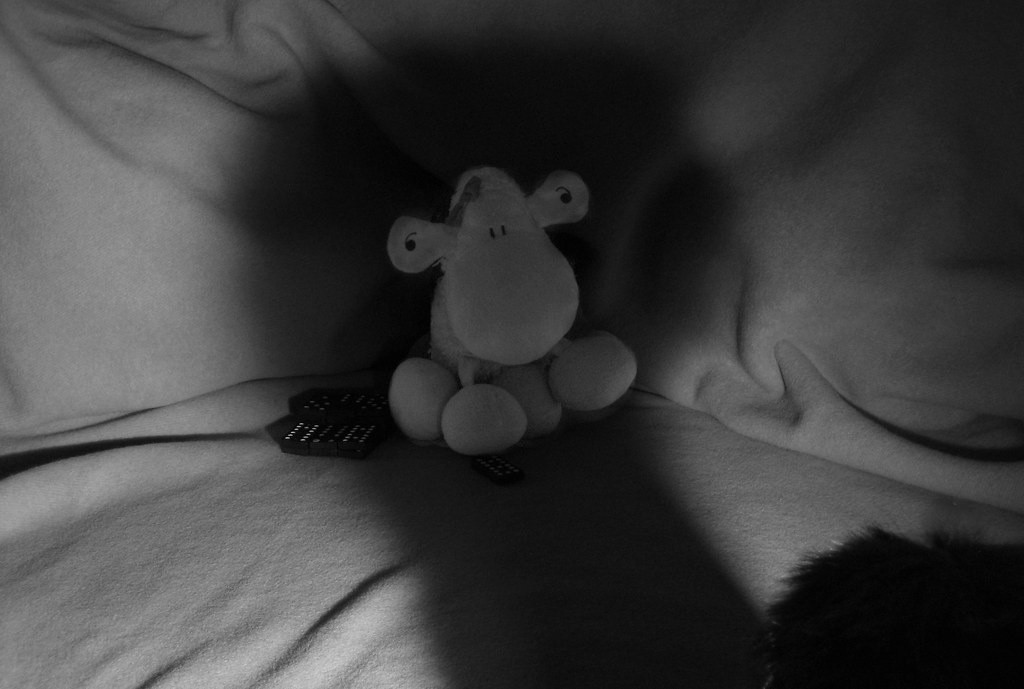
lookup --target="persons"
[759,531,1023,688]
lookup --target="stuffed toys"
[387,163,640,460]
